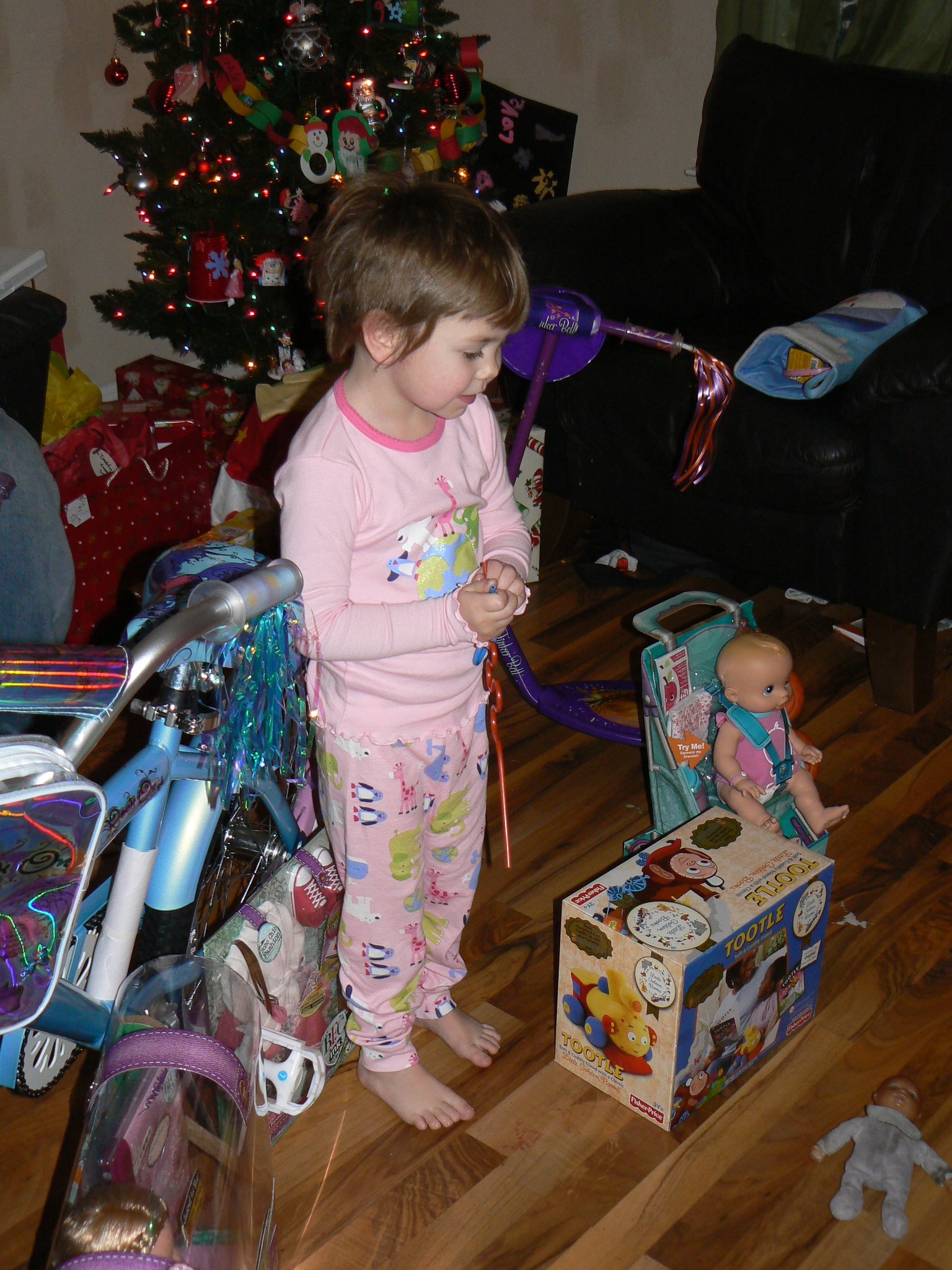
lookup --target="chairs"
[497,33,951,712]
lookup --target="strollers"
[631,590,831,859]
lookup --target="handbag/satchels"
[60,417,216,646]
[0,733,106,1033]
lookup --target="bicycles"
[2,538,344,1106]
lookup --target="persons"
[274,174,533,1131]
[811,1075,952,1239]
[712,634,850,836]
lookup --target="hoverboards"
[499,287,808,748]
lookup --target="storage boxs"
[117,355,236,462]
[554,805,834,1133]
